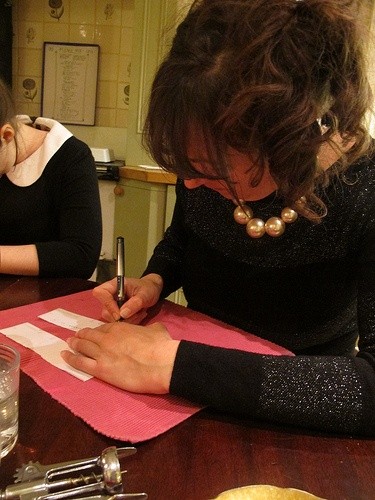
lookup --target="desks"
[0,274,375,500]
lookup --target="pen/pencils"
[112,236,126,323]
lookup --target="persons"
[0,82,103,280]
[60,1,375,438]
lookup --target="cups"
[0,342,20,460]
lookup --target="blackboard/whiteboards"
[40,40,101,127]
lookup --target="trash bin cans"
[85,145,126,265]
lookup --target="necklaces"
[232,196,310,239]
